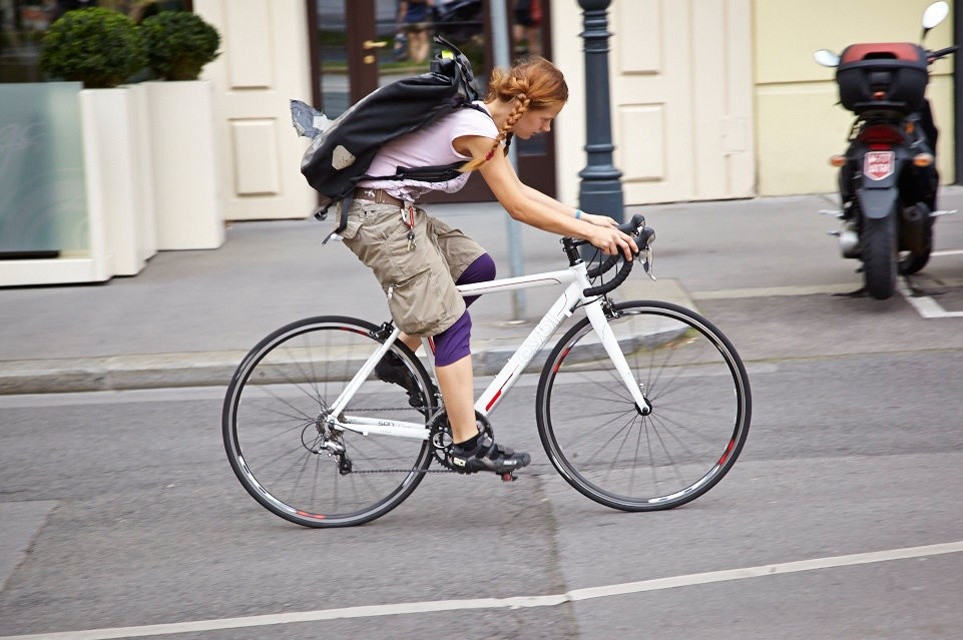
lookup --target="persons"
[335,57,638,479]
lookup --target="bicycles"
[221,214,755,531]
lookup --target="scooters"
[811,2,962,298]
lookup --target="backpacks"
[290,35,494,245]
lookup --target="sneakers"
[375,350,419,392]
[447,432,531,475]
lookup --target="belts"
[345,186,406,208]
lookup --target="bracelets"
[575,210,581,219]
